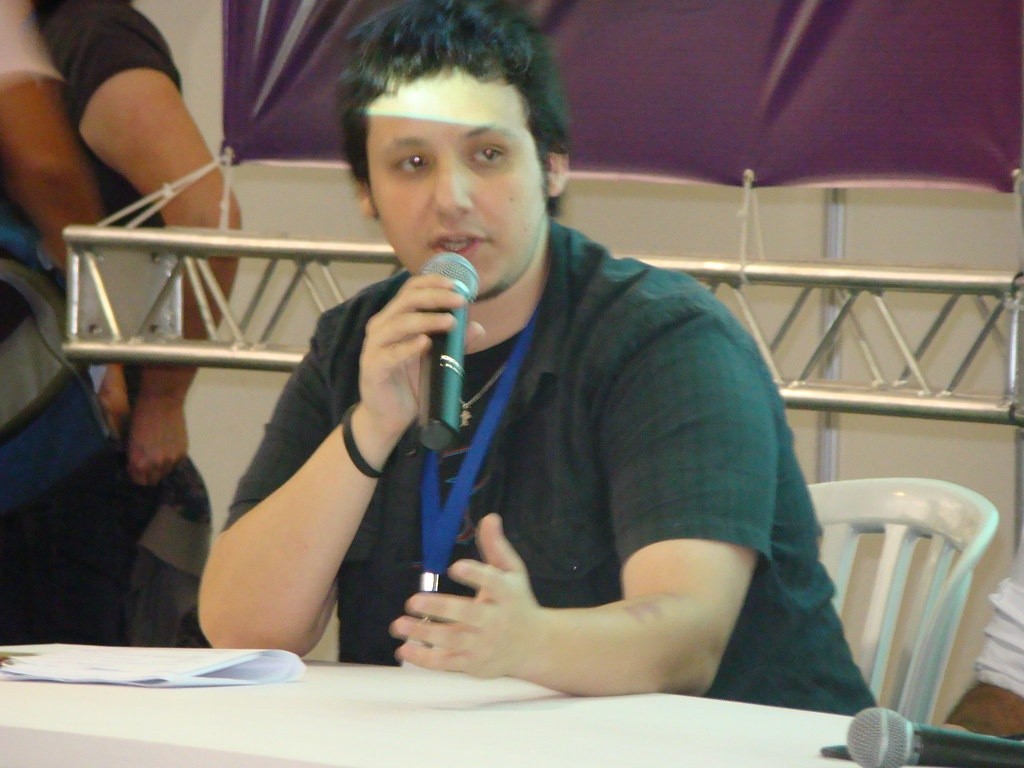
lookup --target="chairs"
[808,477,1001,725]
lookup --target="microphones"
[416,251,481,451]
[846,707,1024,767]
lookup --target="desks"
[0,659,866,768]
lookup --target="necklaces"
[459,360,506,425]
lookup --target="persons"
[937,528,1024,739]
[0,0,241,648]
[197,0,878,717]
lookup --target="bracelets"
[341,401,387,481]
